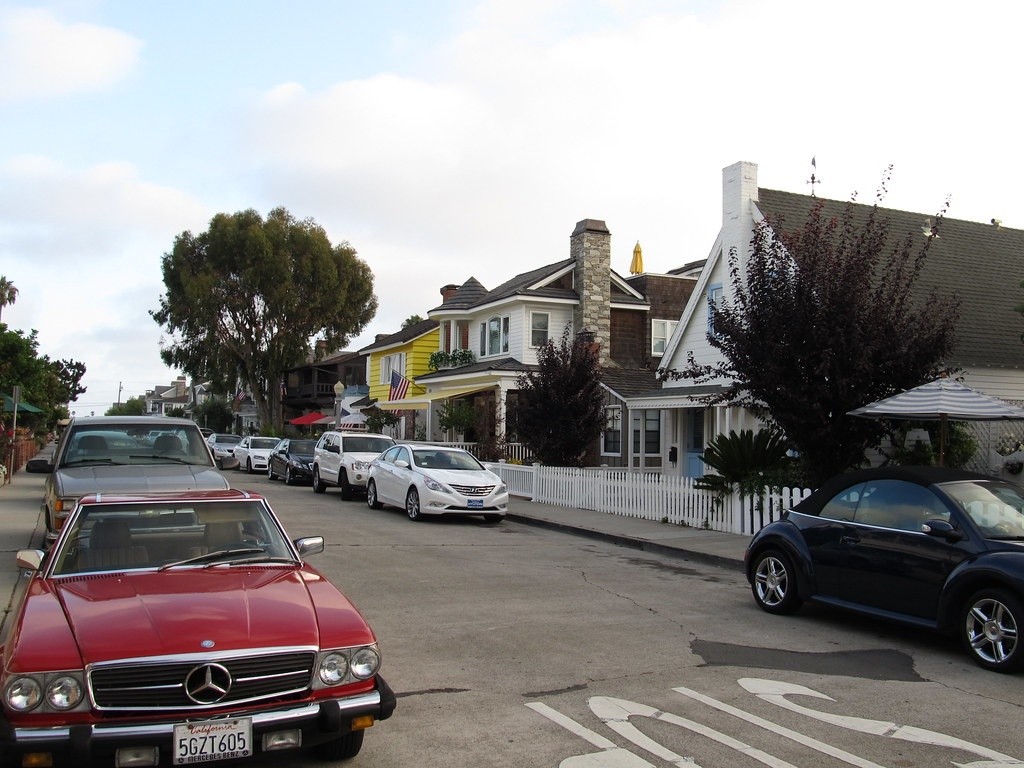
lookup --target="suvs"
[27,414,231,550]
[313,431,402,500]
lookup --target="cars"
[206,434,243,469]
[70,431,140,454]
[267,438,317,485]
[147,426,215,455]
[745,464,1024,673]
[0,489,397,768]
[365,444,509,523]
[232,436,281,474]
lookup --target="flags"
[340,407,355,432]
[388,369,411,416]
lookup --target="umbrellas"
[845,372,1024,467]
[326,410,371,430]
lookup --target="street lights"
[118,382,124,408]
[334,380,344,428]
[9,385,21,484]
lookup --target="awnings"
[290,411,328,424]
[0,391,46,412]
[311,416,336,424]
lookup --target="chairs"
[154,436,185,456]
[75,521,148,570]
[77,435,109,456]
[432,451,453,469]
[187,521,243,558]
[413,454,422,467]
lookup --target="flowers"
[996,432,1024,455]
